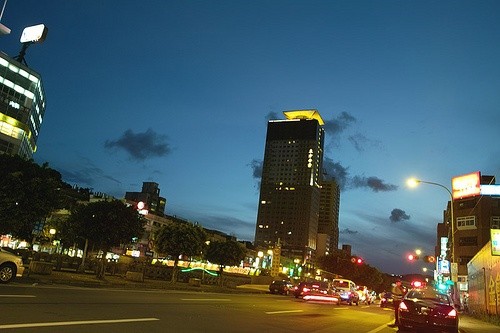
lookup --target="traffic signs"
[144,250,155,257]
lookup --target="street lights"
[407,176,458,298]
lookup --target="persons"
[348,287,357,299]
[387,280,405,327]
[381,290,388,299]
[462,295,467,310]
[363,292,373,305]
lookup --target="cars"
[0,245,26,285]
[397,287,459,333]
[269,276,396,308]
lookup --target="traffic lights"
[137,201,146,210]
[357,259,362,264]
[408,255,421,260]
